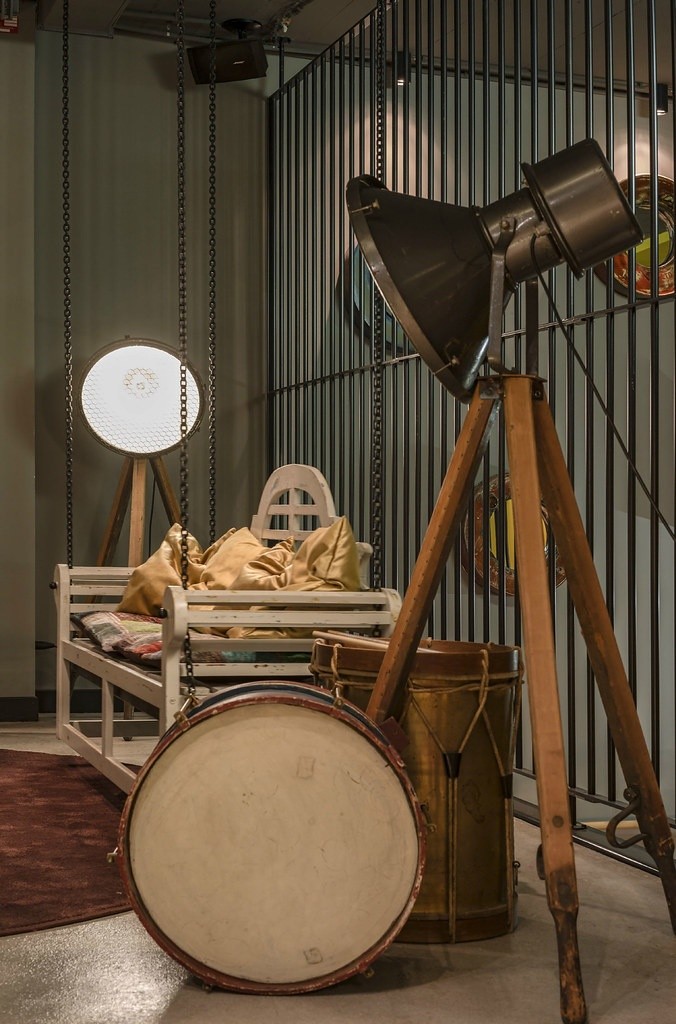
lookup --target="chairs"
[49,464,401,796]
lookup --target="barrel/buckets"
[311,639,524,943]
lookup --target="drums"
[107,679,438,995]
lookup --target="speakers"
[184,37,268,85]
